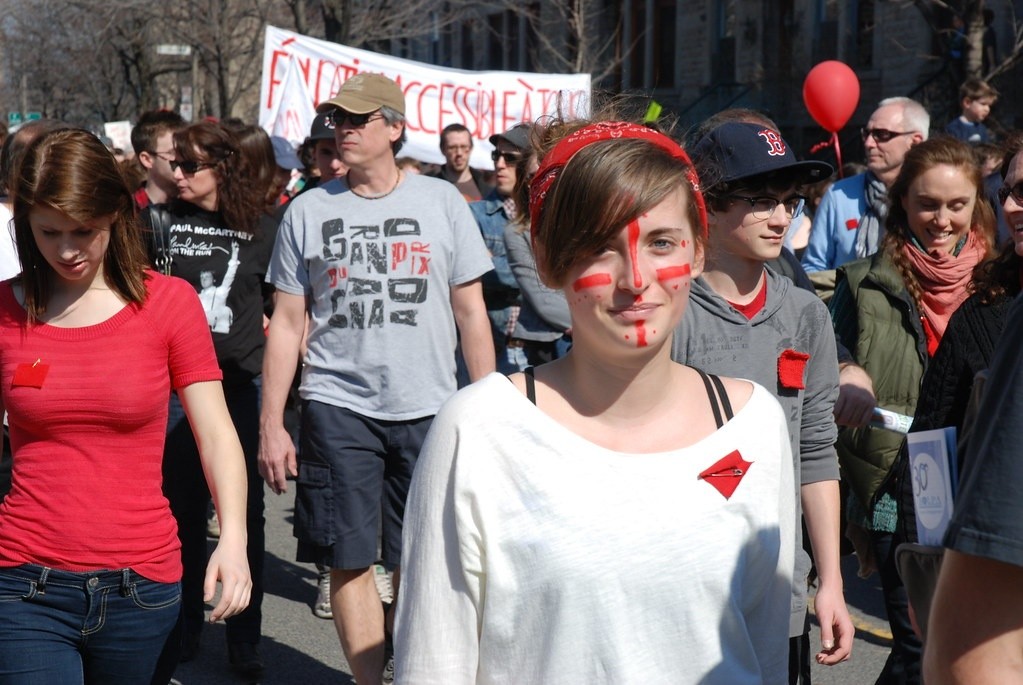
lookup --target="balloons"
[804,60,860,132]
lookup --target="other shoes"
[207,500,221,537]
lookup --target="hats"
[316,72,406,115]
[308,111,336,140]
[270,136,305,169]
[691,121,835,193]
[490,123,546,152]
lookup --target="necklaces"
[346,166,400,200]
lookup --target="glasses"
[859,127,916,142]
[169,160,218,173]
[492,151,523,164]
[998,181,1023,205]
[725,191,809,219]
[330,111,384,126]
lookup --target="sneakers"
[372,564,394,606]
[314,574,334,618]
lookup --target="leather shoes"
[178,632,201,662]
[228,642,265,671]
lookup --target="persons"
[142,122,278,685]
[671,122,857,685]
[391,122,799,685]
[0,130,253,685]
[811,139,998,684]
[922,305,1023,685]
[872,134,1023,685]
[683,77,1023,300]
[435,122,554,381]
[0,111,395,620]
[255,68,498,685]
